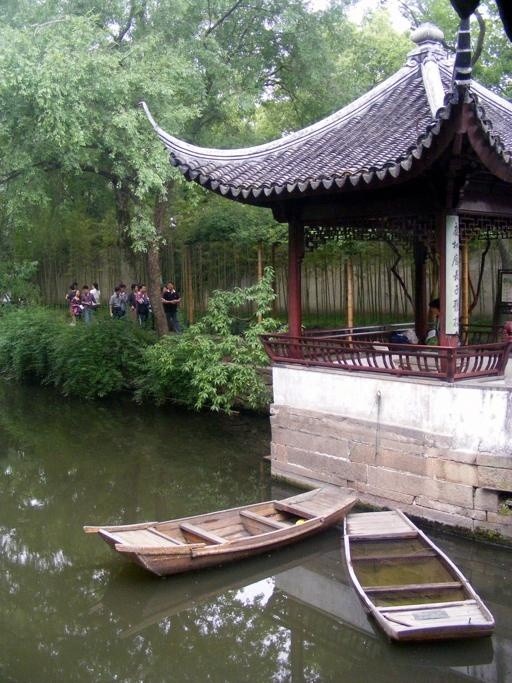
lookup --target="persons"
[63,281,183,334]
[424,299,463,351]
